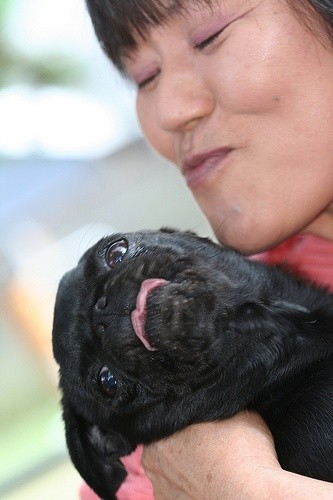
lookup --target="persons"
[81,0,333,499]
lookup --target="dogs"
[53,228,333,500]
